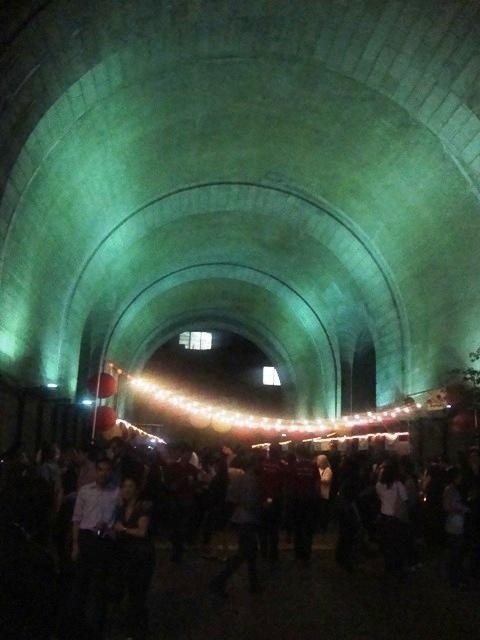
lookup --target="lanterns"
[87,372,117,431]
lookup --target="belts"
[91,528,112,538]
